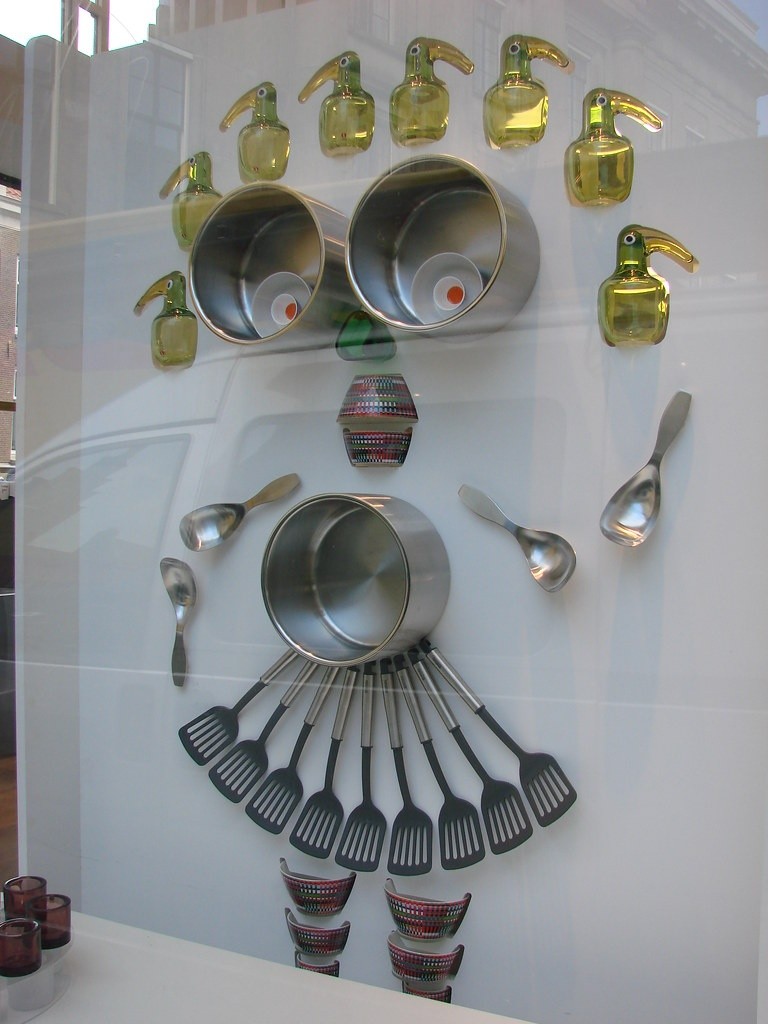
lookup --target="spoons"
[601,391,690,548]
[461,486,575,593]
[178,474,300,552]
[160,558,195,688]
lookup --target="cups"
[26,894,70,949]
[0,918,42,977]
[4,875,46,921]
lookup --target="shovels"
[177,636,578,877]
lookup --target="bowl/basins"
[271,293,298,325]
[251,273,313,337]
[410,253,484,325]
[432,277,466,311]
[346,154,544,341]
[262,494,449,667]
[189,182,354,348]
